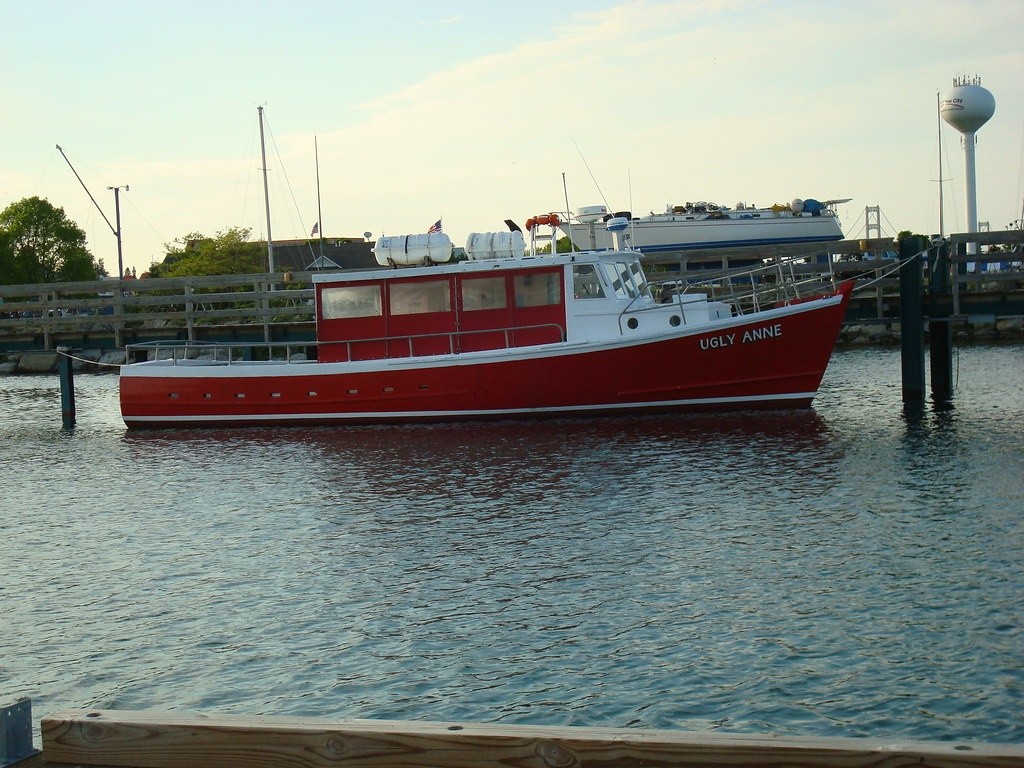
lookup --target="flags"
[428,219,442,234]
[311,222,318,237]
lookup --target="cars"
[0,294,116,320]
[833,249,900,281]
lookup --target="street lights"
[106,183,131,312]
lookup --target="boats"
[547,136,854,252]
[525,212,561,232]
[116,101,862,430]
